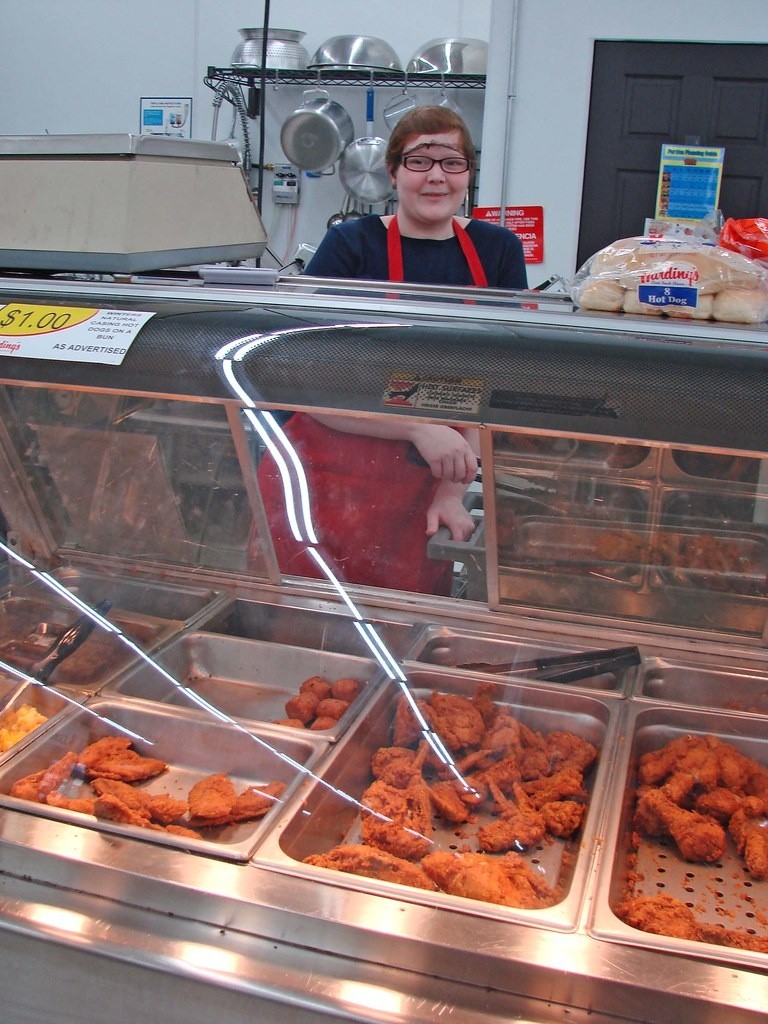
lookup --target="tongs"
[406,440,574,519]
[456,646,642,699]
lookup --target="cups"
[432,93,462,118]
[383,93,419,131]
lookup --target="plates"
[237,28,308,40]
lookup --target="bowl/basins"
[405,39,489,75]
[306,34,404,73]
[230,39,308,69]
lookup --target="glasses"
[399,155,471,174]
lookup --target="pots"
[281,90,353,172]
[339,88,395,204]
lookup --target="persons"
[248,105,531,598]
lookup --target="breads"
[579,236,768,325]
